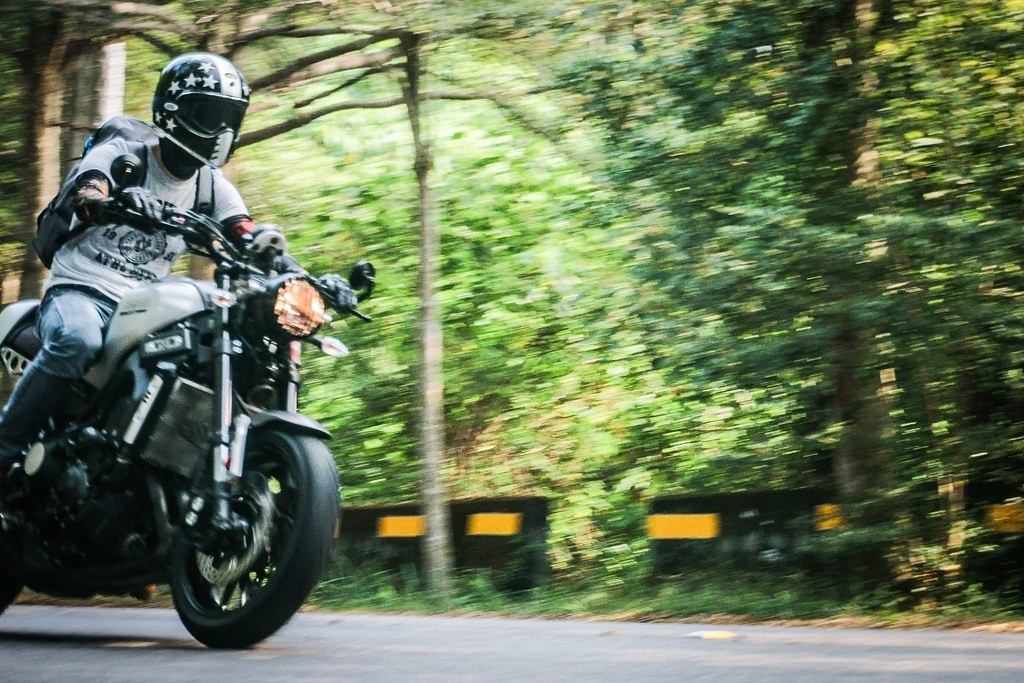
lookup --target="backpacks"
[34,116,215,270]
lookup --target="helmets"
[151,51,249,169]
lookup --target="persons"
[0,52,361,479]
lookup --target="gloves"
[112,186,162,232]
[318,273,357,310]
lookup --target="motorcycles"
[0,151,377,652]
